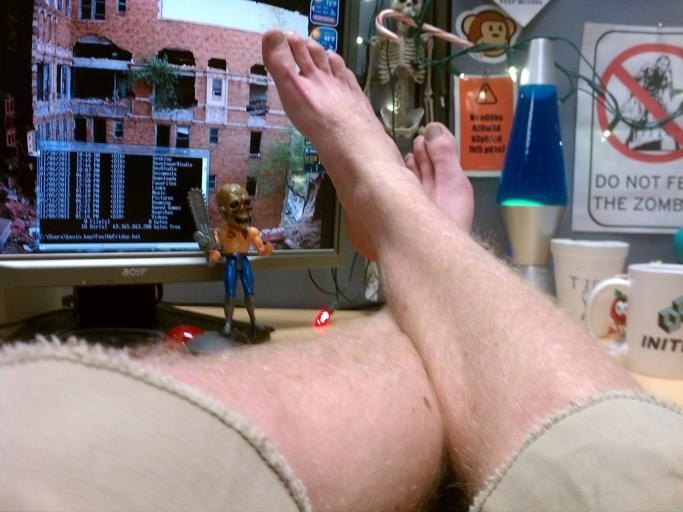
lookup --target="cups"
[585,262,681,382]
[548,233,628,342]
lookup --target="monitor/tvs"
[0,0,360,356]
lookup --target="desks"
[162,302,683,412]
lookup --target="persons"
[193,180,275,336]
[360,0,438,305]
[0,24,683,512]
